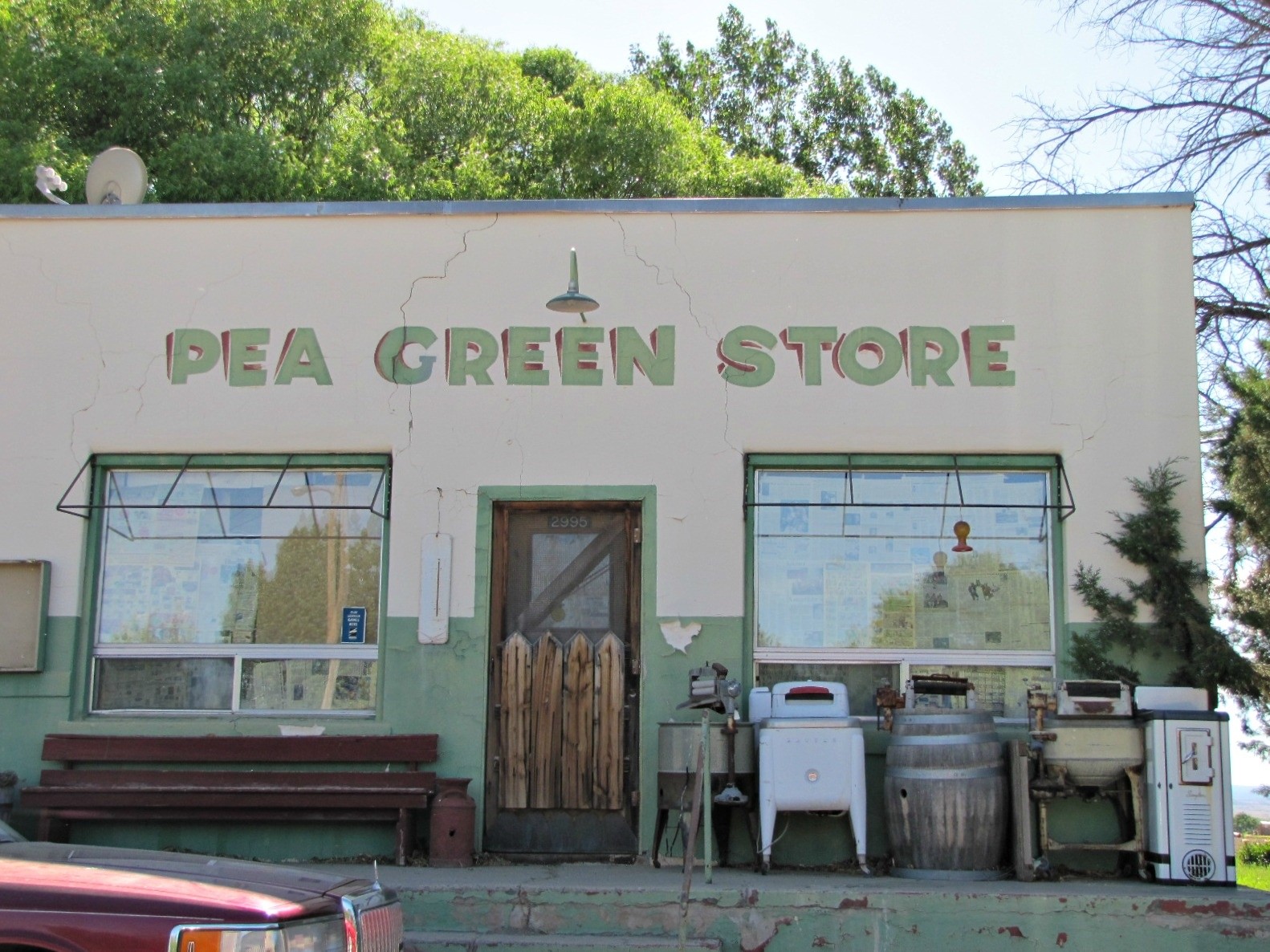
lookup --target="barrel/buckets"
[884,708,1004,881]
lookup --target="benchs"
[22,734,440,866]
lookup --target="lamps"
[546,248,600,322]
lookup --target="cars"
[1,824,406,951]
